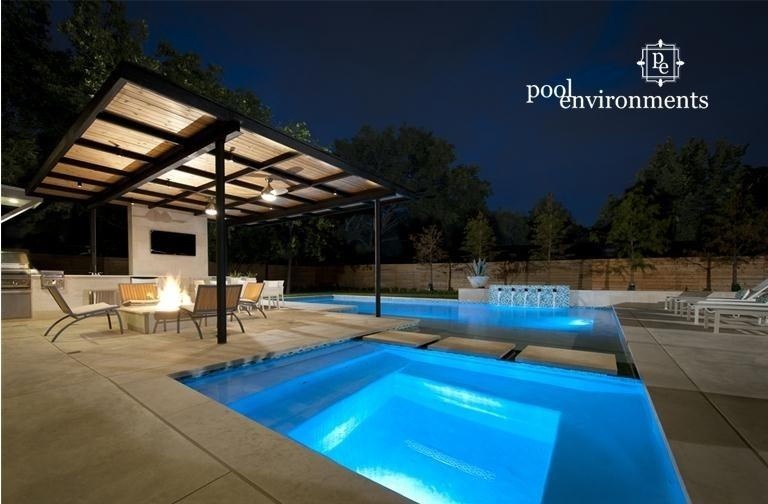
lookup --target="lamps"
[205,196,217,216]
[259,176,288,202]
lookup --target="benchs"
[118,282,163,334]
[176,285,245,340]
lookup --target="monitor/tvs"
[149,229,197,257]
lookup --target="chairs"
[227,275,285,319]
[41,284,124,344]
[663,277,767,333]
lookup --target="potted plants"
[465,255,490,288]
